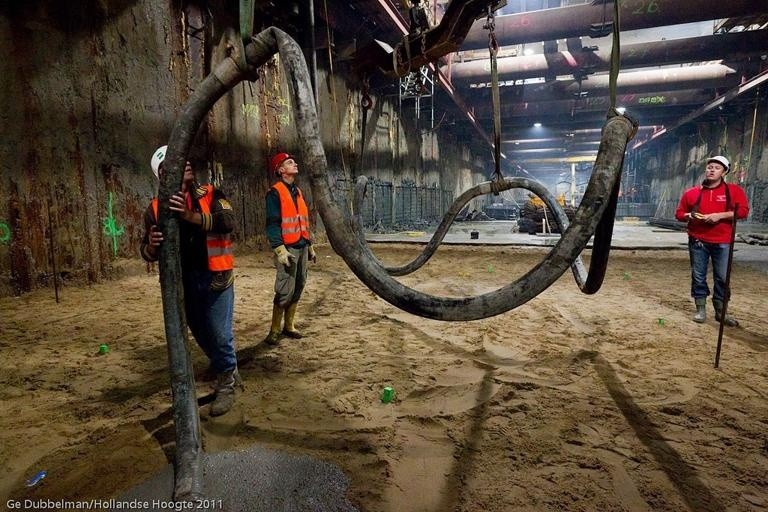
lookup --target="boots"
[693,297,706,323]
[209,366,246,416]
[282,301,302,339]
[263,302,284,345]
[712,298,739,328]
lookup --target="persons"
[675,155,751,326]
[139,143,242,417]
[261,151,316,345]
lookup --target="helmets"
[270,152,294,177]
[151,144,168,181]
[706,156,730,175]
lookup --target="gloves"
[308,245,317,264]
[273,244,296,268]
